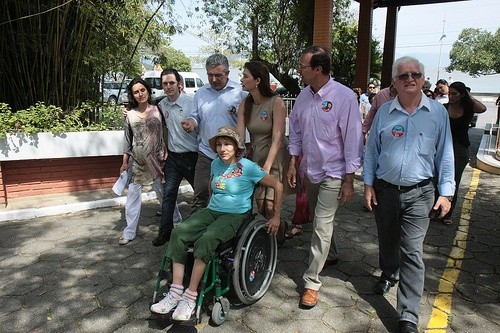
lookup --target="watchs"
[445,196,453,201]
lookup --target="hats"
[207,127,245,157]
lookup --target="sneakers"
[150,292,179,314]
[326,245,339,264]
[170,298,196,321]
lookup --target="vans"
[100,81,129,106]
[143,71,204,105]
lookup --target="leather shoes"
[375,277,392,295]
[399,320,419,333]
[299,289,318,306]
[153,230,171,246]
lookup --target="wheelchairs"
[153,186,278,324]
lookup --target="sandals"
[285,226,304,239]
[119,236,130,245]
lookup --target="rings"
[186,129,189,132]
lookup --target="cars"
[119,82,130,108]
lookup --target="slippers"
[442,217,452,225]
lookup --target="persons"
[149,126,283,322]
[180,54,247,213]
[286,47,365,308]
[353,77,437,128]
[435,78,450,111]
[118,78,183,245]
[286,151,313,237]
[123,69,202,248]
[495,96,500,125]
[363,57,457,333]
[430,82,487,226]
[235,62,288,248]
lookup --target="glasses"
[396,72,423,80]
[369,87,375,89]
